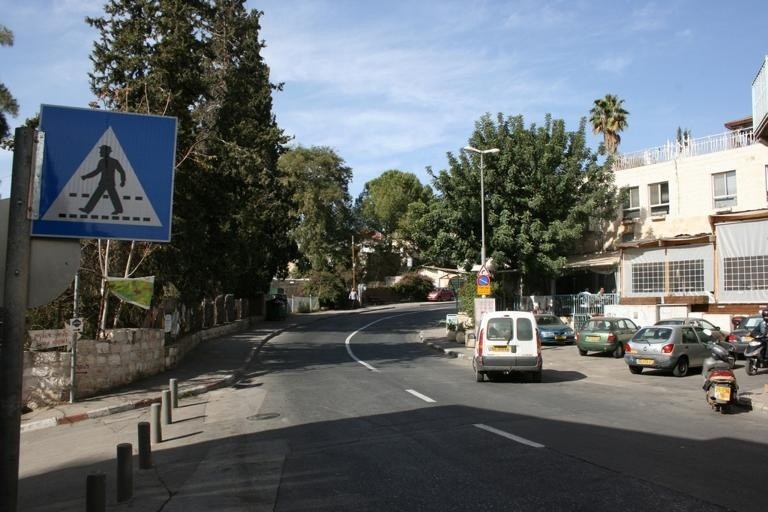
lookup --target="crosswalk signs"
[30,102,176,247]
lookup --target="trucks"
[470,308,542,383]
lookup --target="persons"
[348,287,357,309]
[750,310,768,337]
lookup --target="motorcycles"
[740,323,767,376]
[699,328,741,415]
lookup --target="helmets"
[762,310,768,317]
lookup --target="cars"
[533,312,736,376]
[725,314,765,357]
[427,287,456,301]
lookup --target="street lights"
[461,142,500,312]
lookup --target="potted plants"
[456,323,465,343]
[448,324,456,340]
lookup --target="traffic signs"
[69,317,84,334]
